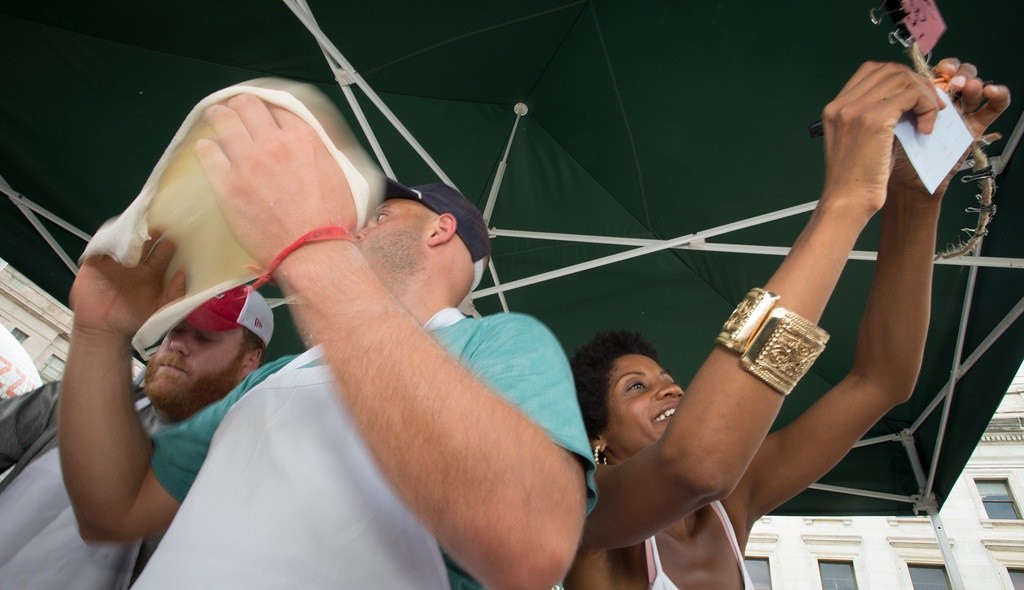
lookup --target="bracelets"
[716,287,830,396]
[247,224,356,291]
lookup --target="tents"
[0,0,1024,590]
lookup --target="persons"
[0,92,597,590]
[562,58,1010,590]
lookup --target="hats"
[382,177,490,293]
[182,283,274,349]
